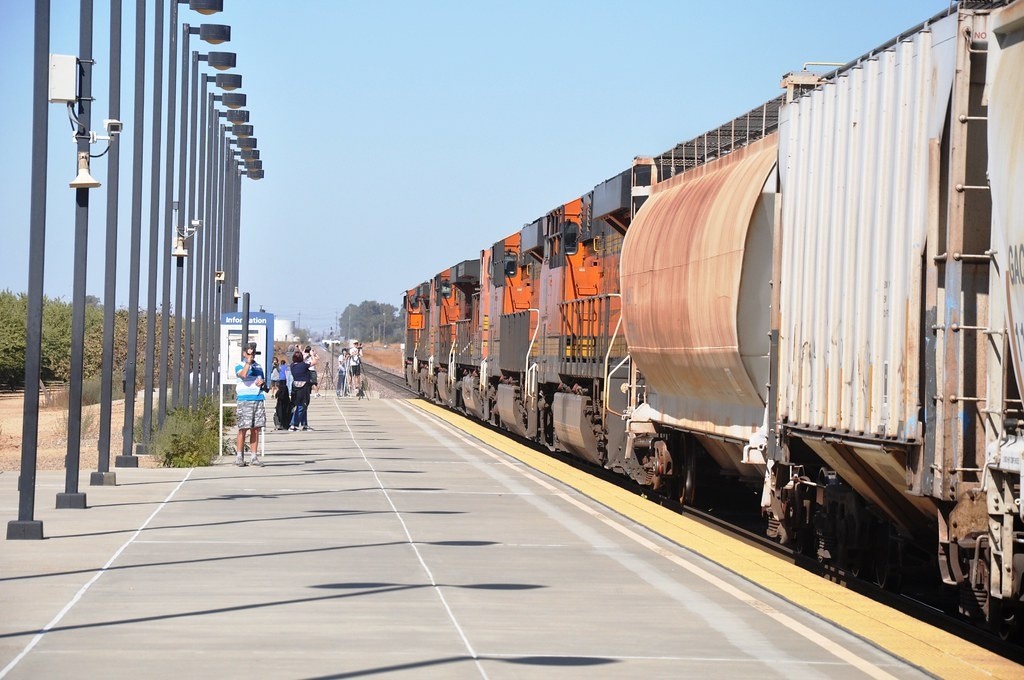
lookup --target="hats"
[353,340,358,343]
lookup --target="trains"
[397,2,1024,658]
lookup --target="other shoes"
[235,459,246,467]
[249,457,264,467]
[301,426,314,432]
[315,393,321,398]
[288,426,300,432]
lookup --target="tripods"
[317,353,371,401]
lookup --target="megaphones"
[215,271,225,280]
[68,153,100,188]
[234,287,241,298]
[172,237,188,258]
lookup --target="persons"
[122,359,127,393]
[336,340,363,397]
[234,343,267,467]
[271,346,322,432]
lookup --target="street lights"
[0,1,268,540]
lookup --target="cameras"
[250,343,261,357]
[305,346,311,353]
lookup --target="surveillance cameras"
[191,219,203,226]
[103,119,123,133]
